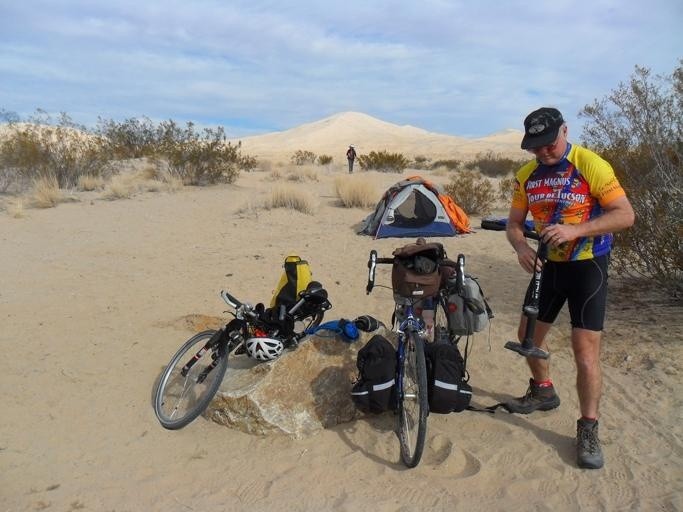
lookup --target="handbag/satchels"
[349,335,398,414]
[445,275,489,335]
[270,255,314,311]
[391,242,444,300]
[426,341,472,414]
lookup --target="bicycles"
[154,281,332,429]
[366,249,466,468]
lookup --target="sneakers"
[507,378,560,414]
[576,418,604,469]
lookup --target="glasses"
[526,144,557,155]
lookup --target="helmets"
[246,338,283,361]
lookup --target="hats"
[520,107,564,149]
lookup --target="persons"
[347,145,356,174]
[506,108,635,469]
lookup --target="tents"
[351,177,474,240]
